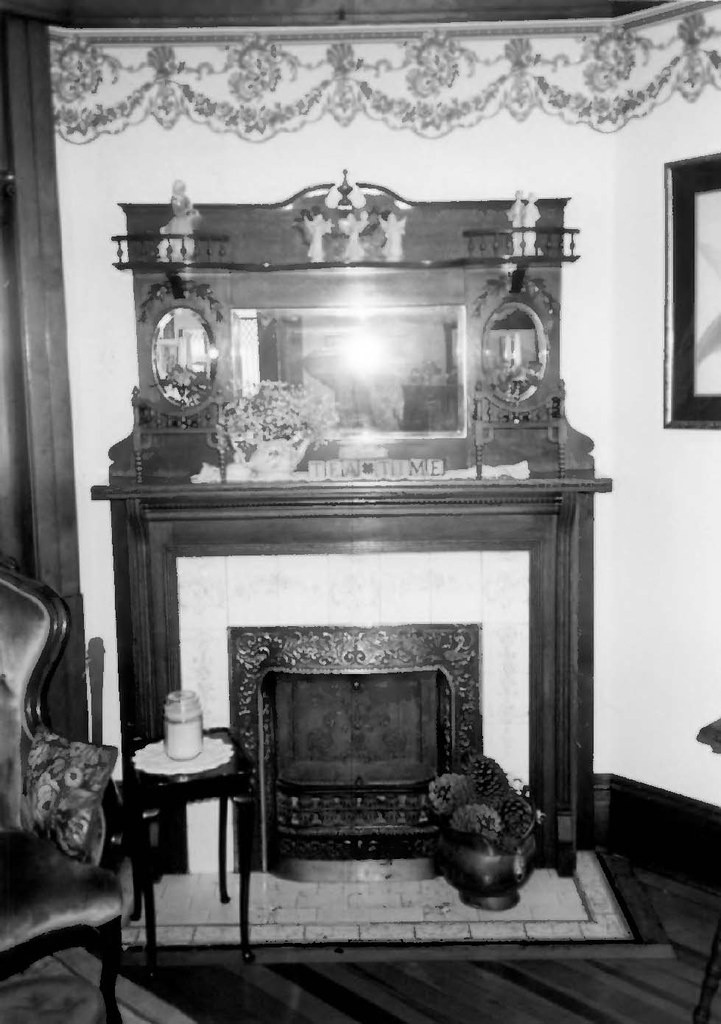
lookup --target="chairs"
[0,550,126,1024]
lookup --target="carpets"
[0,947,198,1024]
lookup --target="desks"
[123,727,255,980]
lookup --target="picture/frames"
[664,152,721,431]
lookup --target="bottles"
[163,690,203,762]
